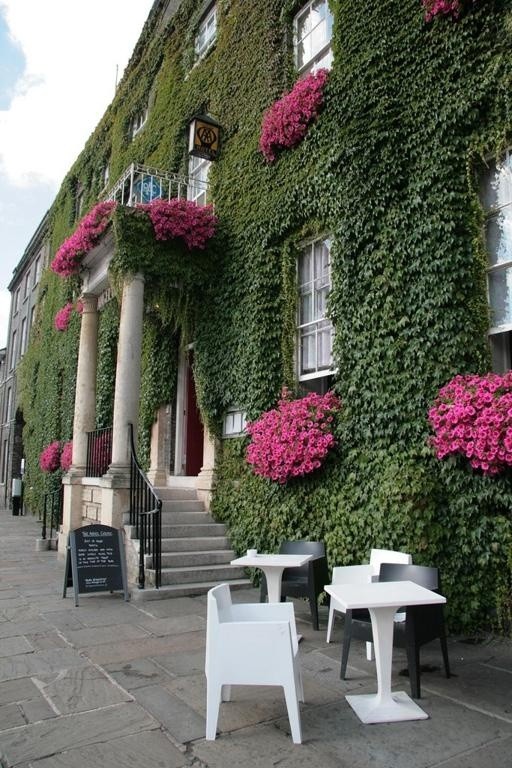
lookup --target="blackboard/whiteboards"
[70,524,128,594]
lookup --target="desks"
[324,580,448,724]
[231,553,314,641]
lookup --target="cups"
[247,550,257,560]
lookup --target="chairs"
[203,582,305,744]
[258,542,330,631]
[326,548,412,662]
[338,563,450,698]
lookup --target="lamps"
[187,114,220,160]
